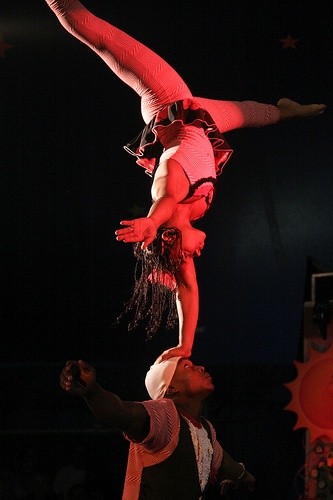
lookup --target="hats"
[145,356,182,400]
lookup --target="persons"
[46,0,325,358]
[55,351,254,499]
